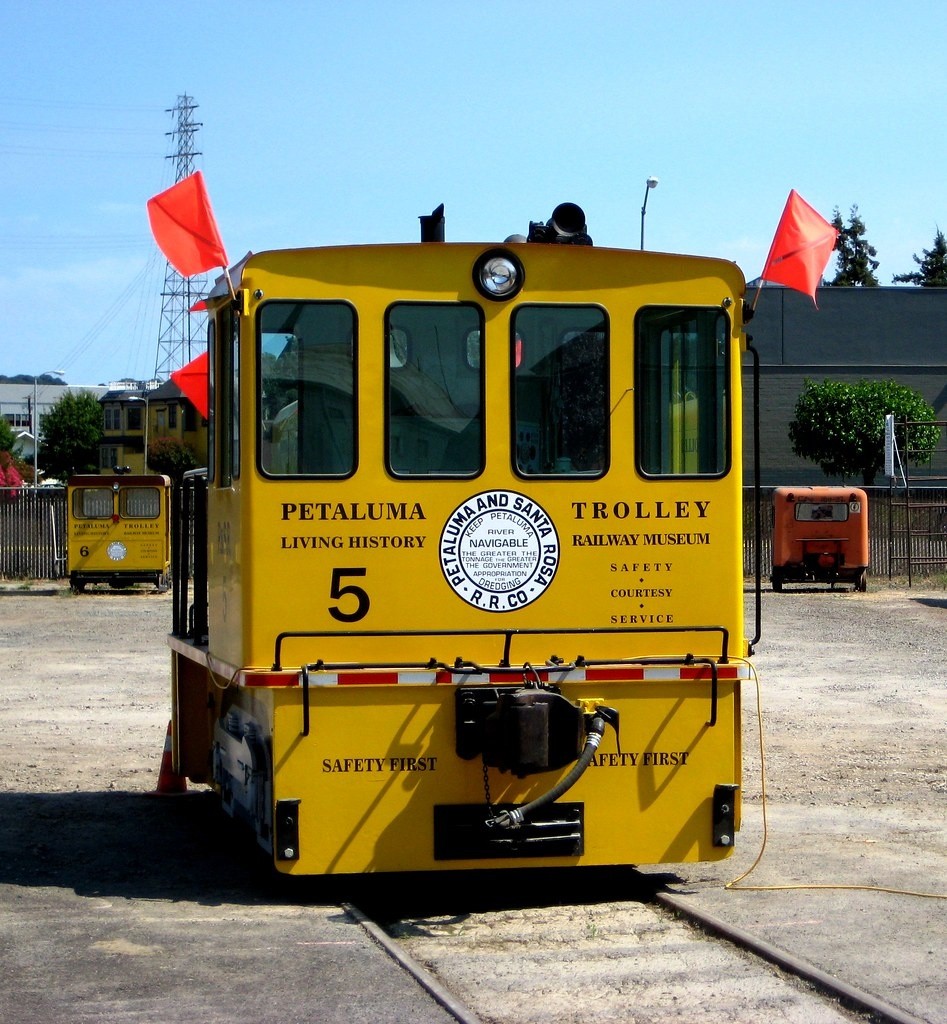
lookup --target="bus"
[368,425,377,430]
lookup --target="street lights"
[640,175,664,251]
[127,395,150,476]
[33,370,65,486]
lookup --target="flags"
[171,354,208,419]
[762,190,840,312]
[148,173,229,277]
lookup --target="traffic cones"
[141,721,202,799]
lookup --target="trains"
[171,199,761,876]
[65,465,171,595]
